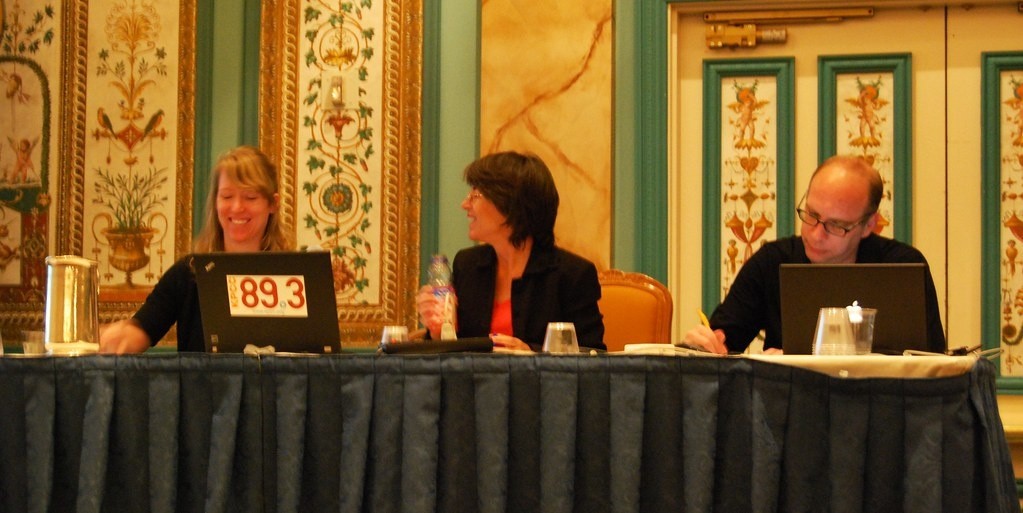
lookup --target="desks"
[0,343,1021,513]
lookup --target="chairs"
[597,269,677,356]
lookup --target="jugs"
[44,256,100,357]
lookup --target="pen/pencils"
[696,308,725,355]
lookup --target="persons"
[99,142,293,357]
[684,156,945,355]
[414,152,608,354]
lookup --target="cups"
[382,326,409,351]
[543,322,579,354]
[812,307,875,355]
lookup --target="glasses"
[468,191,486,203]
[796,190,871,237]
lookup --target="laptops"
[780,263,928,357]
[192,253,343,355]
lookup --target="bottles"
[430,255,454,324]
